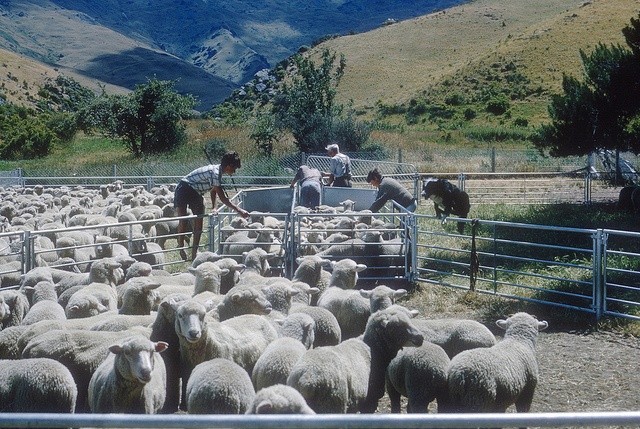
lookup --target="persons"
[324,144,353,187]
[366,168,417,252]
[291,165,324,210]
[174,152,251,262]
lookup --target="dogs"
[421,177,471,234]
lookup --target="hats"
[366,168,379,183]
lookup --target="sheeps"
[0,247,548,415]
[0,180,192,253]
[221,199,411,277]
